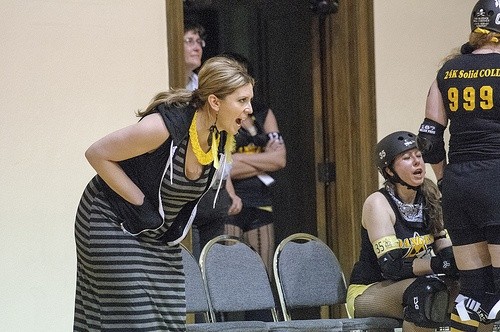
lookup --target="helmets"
[373,131,417,180]
[470,0,500,33]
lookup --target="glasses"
[183,38,205,47]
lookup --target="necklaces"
[189,111,221,165]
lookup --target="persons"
[345,130,459,332]
[72,56,254,332]
[183,18,287,284]
[417,0,500,332]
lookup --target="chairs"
[199,235,343,332]
[179,243,270,332]
[273,233,403,332]
